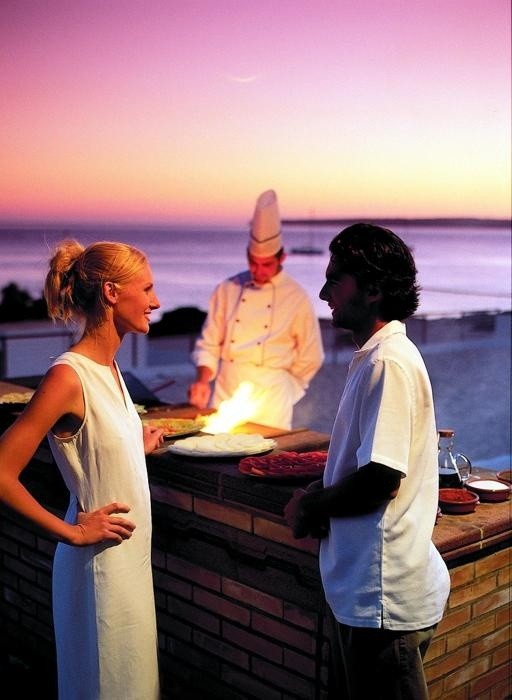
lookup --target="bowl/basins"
[464,478,511,502]
[439,489,480,513]
[496,469,512,483]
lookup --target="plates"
[142,418,206,438]
[166,438,278,458]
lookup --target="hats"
[248,189,286,258]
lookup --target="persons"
[188,247,326,431]
[0,238,167,700]
[282,221,455,699]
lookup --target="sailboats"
[290,206,325,256]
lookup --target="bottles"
[437,430,471,488]
